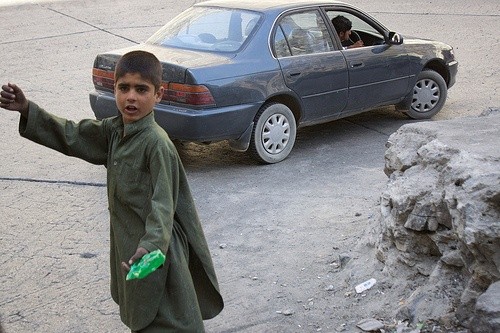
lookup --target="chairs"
[196,33,215,44]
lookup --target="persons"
[330,14,364,52]
[0,48,225,333]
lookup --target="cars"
[89,1,458,164]
